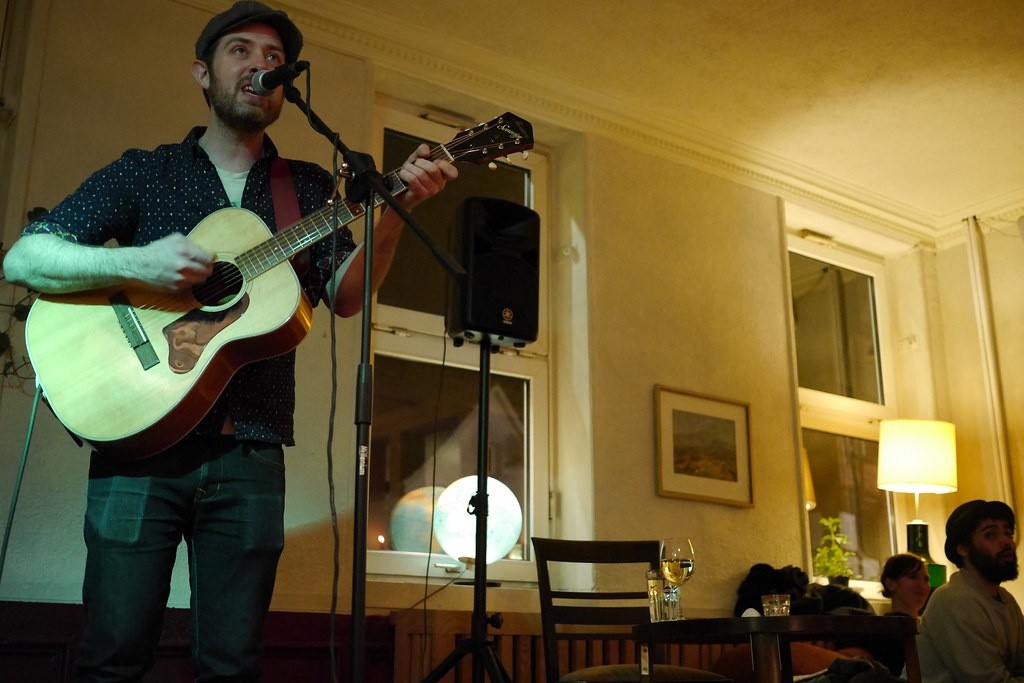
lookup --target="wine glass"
[660,537,695,620]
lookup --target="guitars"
[26,112,533,459]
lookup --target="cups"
[645,569,673,623]
[762,593,790,617]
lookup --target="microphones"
[252,60,310,95]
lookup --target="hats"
[944,500,1015,568]
[195,1,303,69]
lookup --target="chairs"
[532,537,667,682]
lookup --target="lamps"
[877,418,958,615]
[432,475,523,587]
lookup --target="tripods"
[423,338,511,683]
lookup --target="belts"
[190,411,236,437]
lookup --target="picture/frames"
[652,382,755,508]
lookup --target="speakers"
[445,196,541,347]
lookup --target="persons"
[897,499,1024,682]
[879,553,931,677]
[1,4,460,683]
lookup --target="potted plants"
[813,517,855,587]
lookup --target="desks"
[628,610,920,682]
